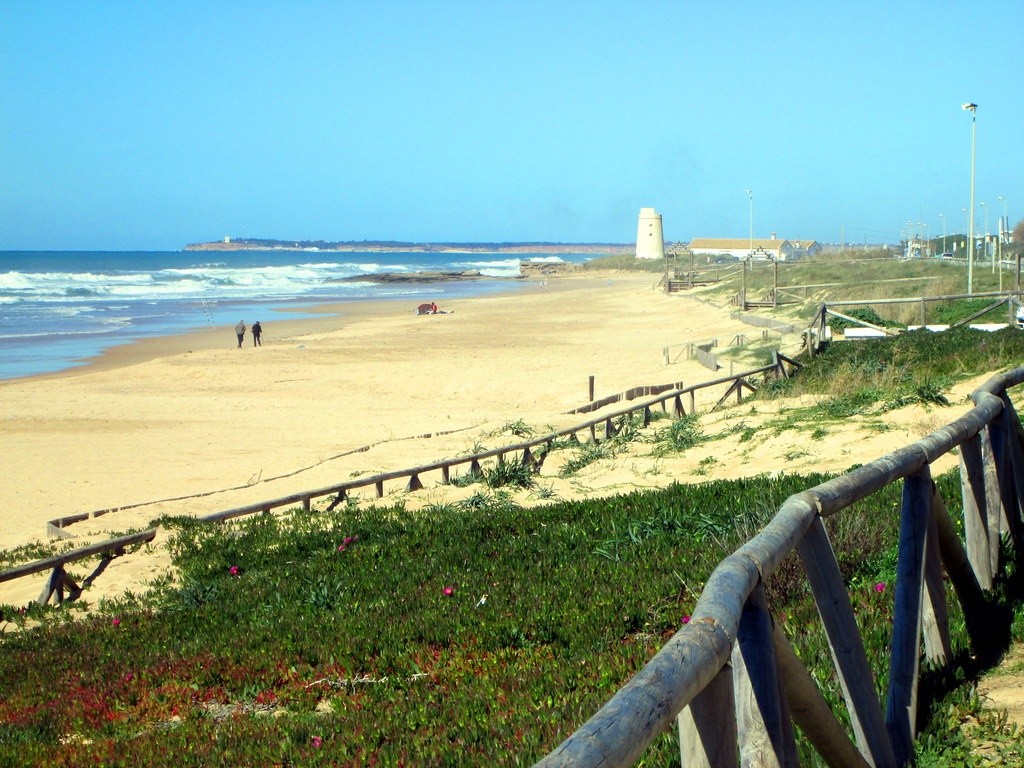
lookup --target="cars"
[939,253,955,260]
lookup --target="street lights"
[962,208,969,263]
[746,188,754,269]
[938,214,946,254]
[979,202,988,260]
[962,102,980,301]
[998,196,1007,244]
[922,223,930,258]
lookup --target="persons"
[431,302,437,314]
[540,279,548,292]
[235,320,246,347]
[252,321,262,347]
